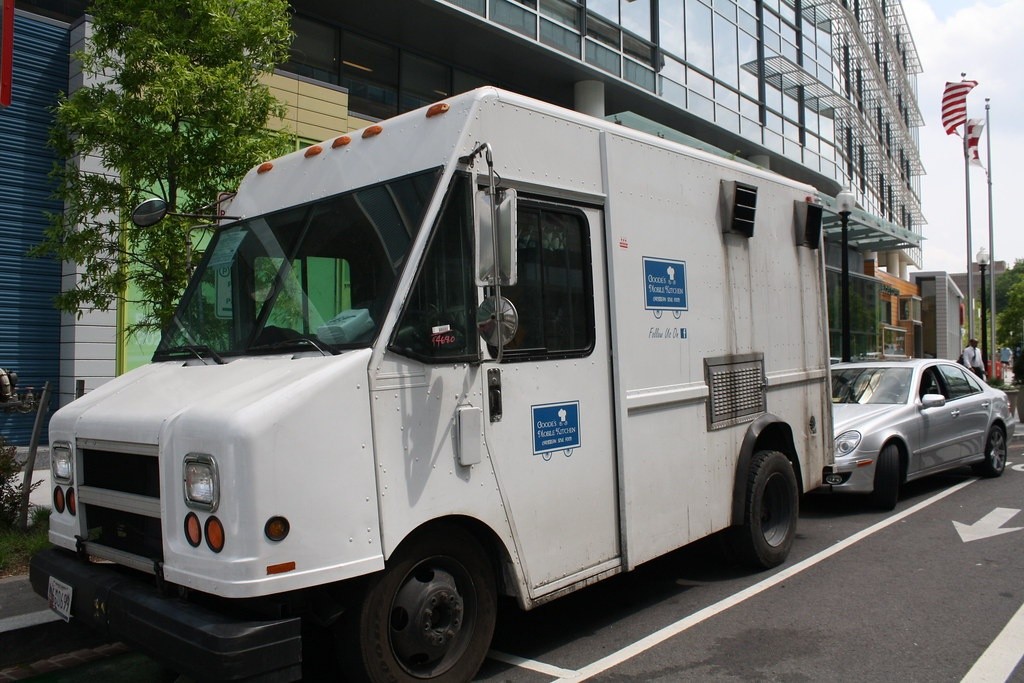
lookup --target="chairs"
[919,372,931,398]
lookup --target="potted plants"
[983,376,1019,419]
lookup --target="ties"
[972,347,976,362]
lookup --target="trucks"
[29,84,836,683]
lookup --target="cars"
[806,357,1016,513]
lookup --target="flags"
[967,120,984,169]
[941,81,979,136]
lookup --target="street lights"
[836,183,857,363]
[976,245,992,380]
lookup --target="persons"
[996,344,1011,378]
[963,339,987,380]
